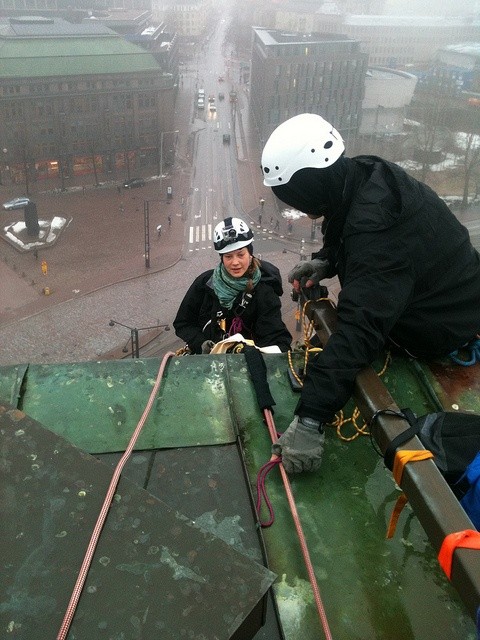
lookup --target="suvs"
[198,88,205,98]
[210,103,216,110]
[197,98,204,108]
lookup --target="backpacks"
[384,408,479,530]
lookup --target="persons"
[172,217,294,356]
[258,197,293,233]
[156,214,173,236]
[33,248,39,265]
[261,113,480,474]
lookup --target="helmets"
[213,218,253,254]
[261,113,346,186]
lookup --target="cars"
[208,94,215,102]
[122,178,144,189]
[218,75,225,81]
[218,92,224,99]
[223,134,230,143]
[2,196,30,210]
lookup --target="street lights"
[160,130,179,193]
